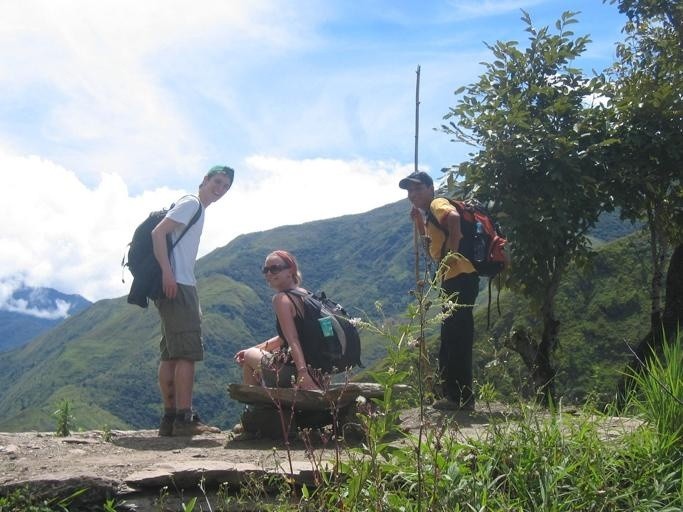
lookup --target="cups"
[317,316,334,337]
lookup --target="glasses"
[260,264,290,274]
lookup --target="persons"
[397,169,480,412]
[150,164,234,434]
[228,248,332,436]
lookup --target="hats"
[199,166,234,187]
[399,172,433,189]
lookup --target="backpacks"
[281,288,360,373]
[128,195,202,300]
[428,197,508,276]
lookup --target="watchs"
[296,367,307,375]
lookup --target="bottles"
[474,222,487,263]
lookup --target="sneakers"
[232,423,245,432]
[159,416,221,436]
[433,398,474,410]
